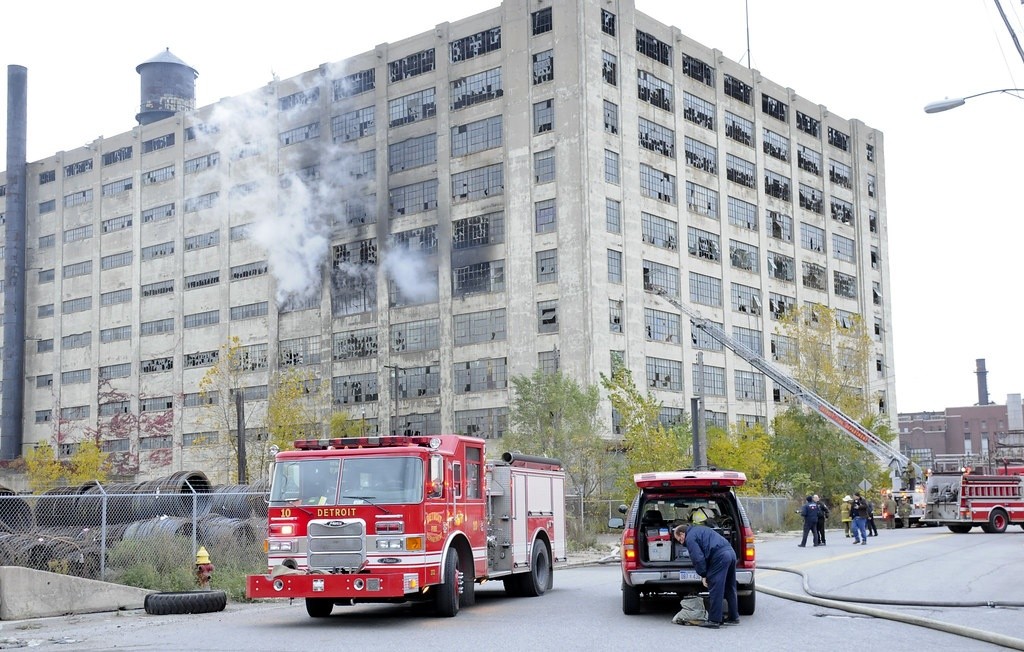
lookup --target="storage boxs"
[648,540,672,561]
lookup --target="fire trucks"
[647,279,1024,535]
[247,433,566,618]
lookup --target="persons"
[849,494,868,545]
[884,494,897,529]
[902,460,915,490]
[798,496,820,547]
[861,496,878,536]
[674,525,740,629]
[898,498,912,529]
[812,495,829,545]
[840,496,854,538]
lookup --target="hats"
[843,495,853,502]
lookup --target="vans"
[607,463,758,616]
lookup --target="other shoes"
[845,535,850,537]
[813,544,818,547]
[798,544,805,547]
[868,534,873,536]
[851,535,854,537]
[861,541,866,545]
[853,540,860,544]
[874,534,878,536]
[819,543,826,546]
[699,621,719,629]
[724,618,740,624]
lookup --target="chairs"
[643,510,663,520]
